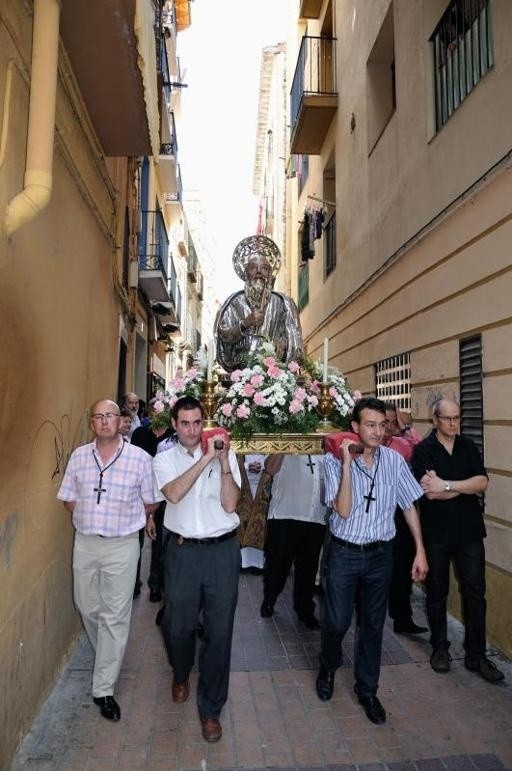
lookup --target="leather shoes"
[316,664,338,703]
[201,717,224,743]
[357,692,387,726]
[92,696,121,721]
[171,676,190,705]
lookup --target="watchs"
[400,424,411,434]
[445,480,451,491]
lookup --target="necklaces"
[354,446,380,513]
[93,440,125,504]
[307,454,315,474]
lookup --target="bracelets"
[221,471,231,475]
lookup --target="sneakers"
[297,610,322,631]
[430,647,451,674]
[260,594,277,620]
[133,579,163,603]
[393,617,429,636]
[465,654,506,682]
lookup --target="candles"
[322,338,328,382]
[207,340,213,382]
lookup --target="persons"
[236,454,273,575]
[316,398,429,724]
[217,254,304,372]
[153,398,242,743]
[381,403,428,635]
[412,398,505,683]
[56,400,165,720]
[261,453,333,629]
[119,393,205,639]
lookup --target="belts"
[162,525,237,546]
[330,535,392,550]
